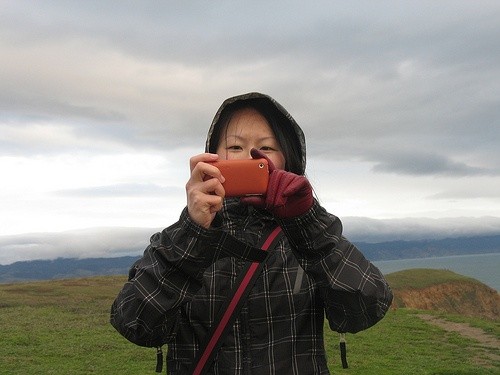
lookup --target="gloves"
[240,148,313,216]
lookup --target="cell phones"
[203,158,268,197]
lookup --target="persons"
[110,92,394,375]
